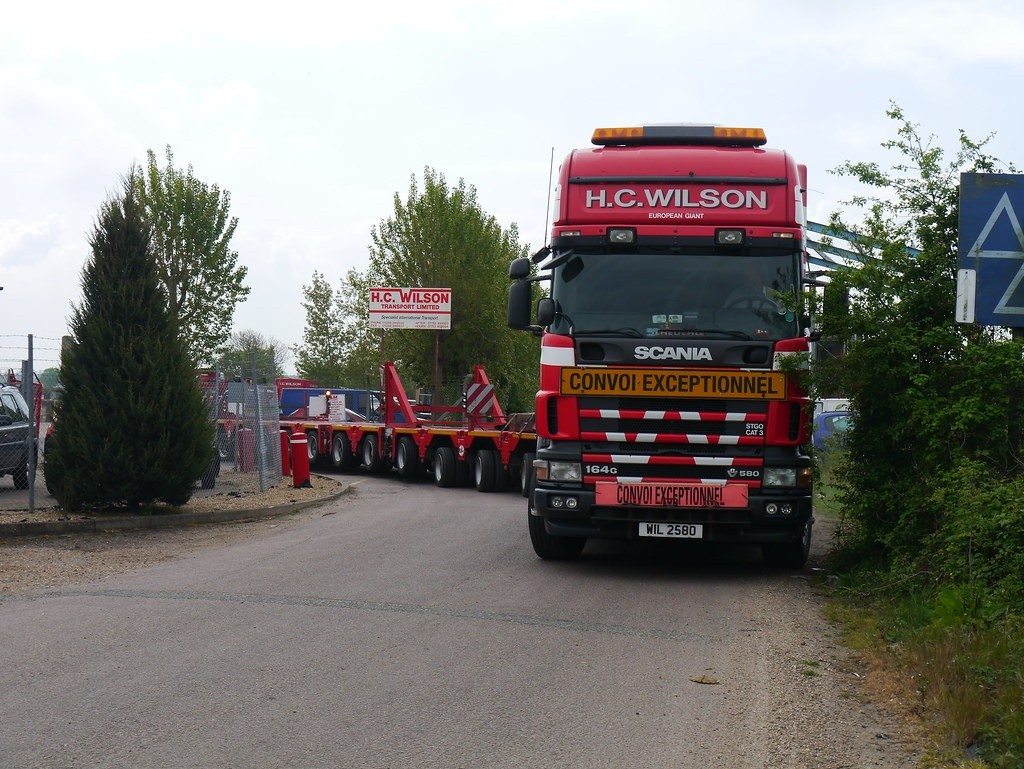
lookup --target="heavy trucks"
[189,363,320,462]
[215,125,851,573]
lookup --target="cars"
[393,399,432,422]
[280,387,407,424]
[369,395,385,412]
[44,402,64,459]
[811,410,862,452]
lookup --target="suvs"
[0,382,39,490]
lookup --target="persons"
[725,259,783,308]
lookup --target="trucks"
[813,399,851,419]
